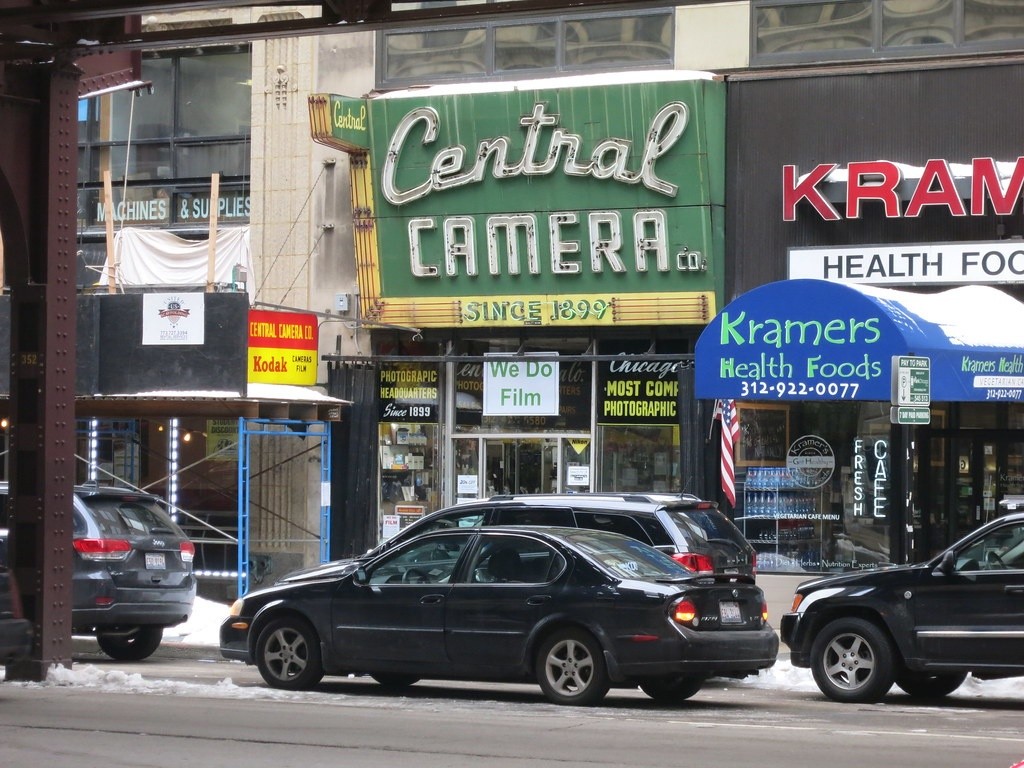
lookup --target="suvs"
[273,491,767,702]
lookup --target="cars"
[781,515,1024,701]
[220,501,780,705]
[0,482,195,667]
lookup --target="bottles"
[378,422,427,470]
[744,466,823,571]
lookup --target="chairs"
[485,549,523,584]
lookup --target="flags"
[714,398,741,509]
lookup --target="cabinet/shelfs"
[742,482,823,571]
[382,442,429,472]
